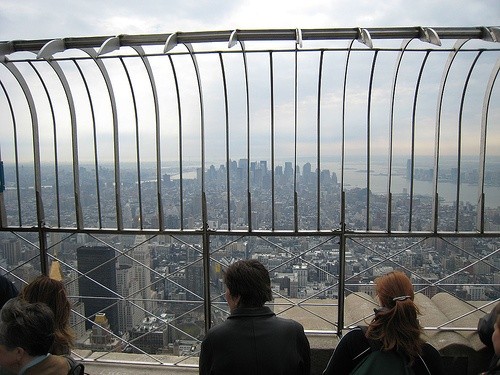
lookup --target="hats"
[478,302,500,346]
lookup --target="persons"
[17,275,77,355]
[198,257,313,375]
[478,300,500,375]
[321,271,443,375]
[0,298,89,375]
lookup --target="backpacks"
[63,354,85,375]
[350,325,415,375]
